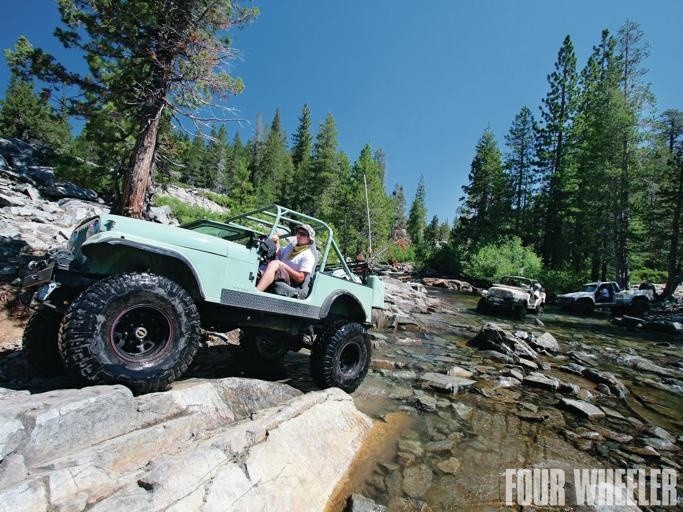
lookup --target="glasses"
[296,231,308,237]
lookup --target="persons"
[596,286,610,303]
[257,224,316,291]
[533,283,542,315]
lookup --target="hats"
[294,224,315,241]
[535,284,541,289]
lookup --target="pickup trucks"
[559,281,654,313]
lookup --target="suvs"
[477,276,547,318]
[21,204,386,395]
